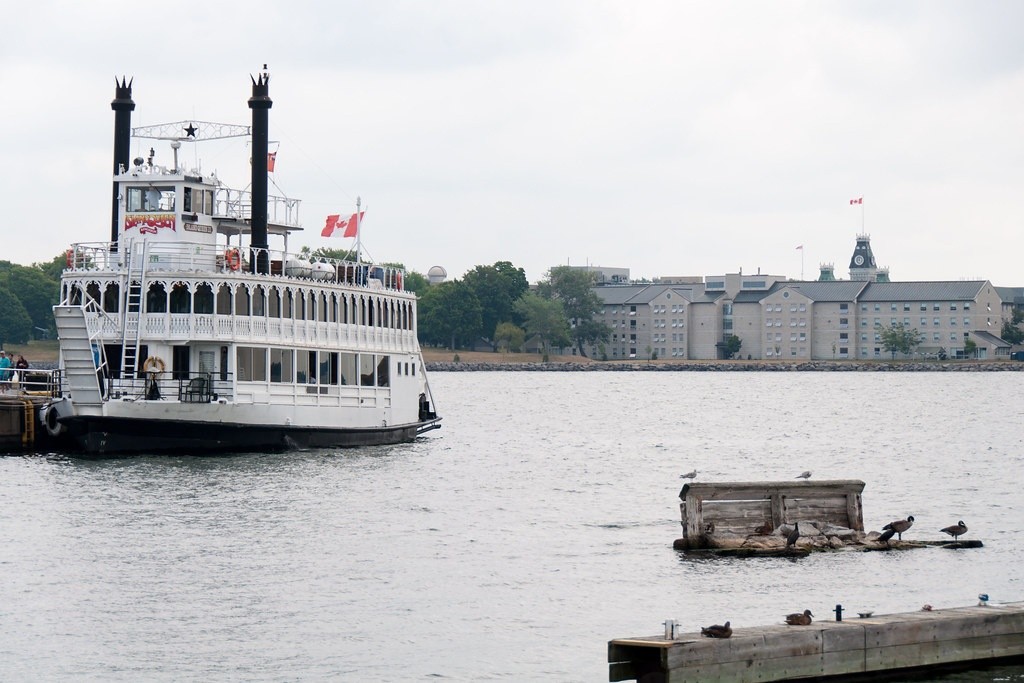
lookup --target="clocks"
[870,256,875,265]
[854,255,864,266]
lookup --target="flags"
[849,197,864,205]
[320,213,364,239]
[796,245,803,249]
[267,152,277,173]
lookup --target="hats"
[0,351,5,354]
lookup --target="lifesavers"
[142,355,166,376]
[397,272,402,290]
[227,250,240,270]
[36,402,62,438]
[66,248,82,268]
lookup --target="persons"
[16,355,29,389]
[6,352,15,389]
[0,351,11,393]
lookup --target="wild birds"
[782,609,814,625]
[797,471,812,481]
[756,522,772,537]
[870,516,914,546]
[780,520,799,546]
[939,521,968,541]
[700,621,733,638]
[680,469,696,482]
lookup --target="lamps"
[134,157,145,167]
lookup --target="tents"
[913,346,946,361]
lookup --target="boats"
[52,64,442,451]
